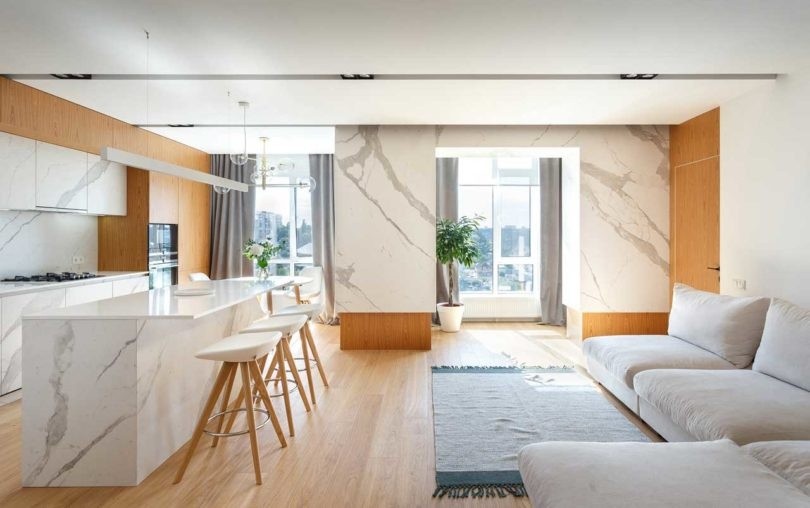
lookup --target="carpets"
[431,365,654,499]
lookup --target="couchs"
[518,282,810,508]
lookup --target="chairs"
[286,266,325,324]
[189,272,210,282]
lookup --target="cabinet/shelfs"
[0,132,127,215]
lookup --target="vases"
[254,267,271,285]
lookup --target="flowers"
[241,237,287,278]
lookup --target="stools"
[223,314,311,437]
[256,304,329,405]
[171,332,287,486]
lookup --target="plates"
[172,288,217,297]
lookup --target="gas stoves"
[1,271,107,282]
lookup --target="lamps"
[213,102,317,194]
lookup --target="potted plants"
[437,213,487,332]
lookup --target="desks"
[20,277,295,487]
